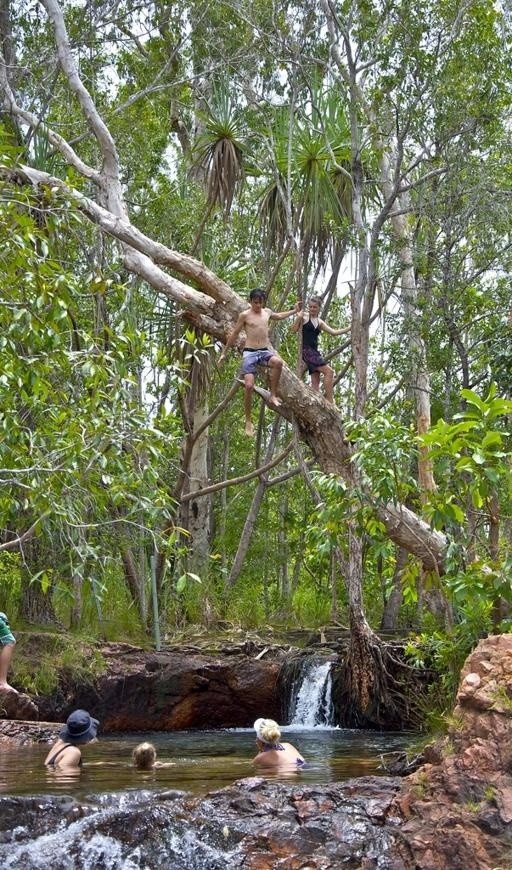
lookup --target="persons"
[217,288,300,438]
[290,295,353,404]
[131,741,162,771]
[249,718,307,768]
[44,709,100,766]
[0,612,20,694]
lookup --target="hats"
[59,709,101,744]
[253,717,281,744]
